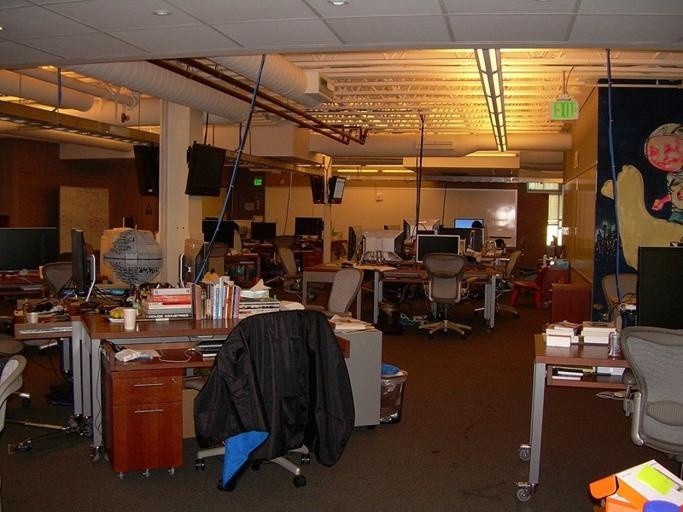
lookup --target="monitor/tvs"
[251,222,276,240]
[185,141,227,197]
[0,227,58,275]
[71,228,85,293]
[295,217,322,234]
[134,144,159,196]
[636,246,683,329]
[311,174,325,204]
[348,218,484,264]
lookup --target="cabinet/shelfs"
[516,334,639,502]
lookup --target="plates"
[105,315,124,322]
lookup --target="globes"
[104,230,163,309]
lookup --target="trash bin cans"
[378,302,401,329]
[380,363,408,425]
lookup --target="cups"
[26,312,39,325]
[122,309,137,331]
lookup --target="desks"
[538,266,592,324]
[14,295,383,481]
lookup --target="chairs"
[1,355,27,432]
[264,248,525,341]
[620,326,683,479]
[194,308,355,490]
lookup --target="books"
[141,274,281,324]
[328,314,376,335]
[542,318,627,382]
